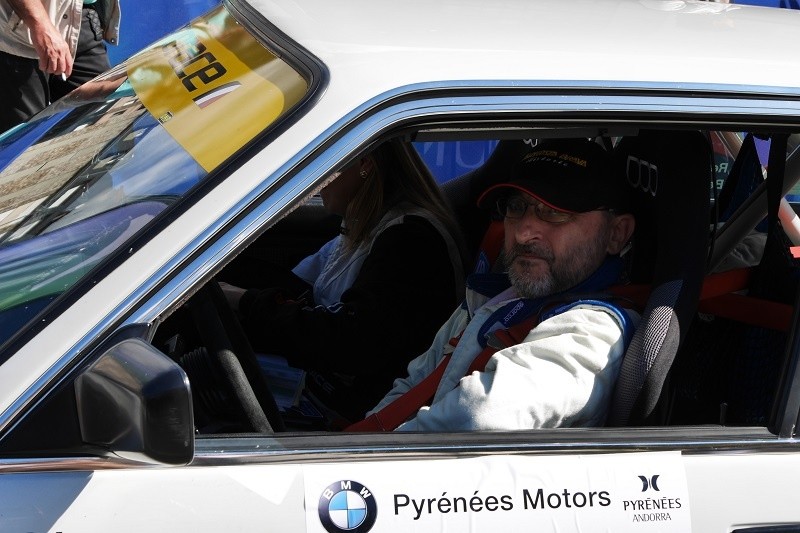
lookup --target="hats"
[476,138,633,216]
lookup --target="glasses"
[496,194,612,225]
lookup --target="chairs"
[605,274,708,430]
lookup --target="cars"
[2,0,800,533]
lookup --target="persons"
[0,0,120,143]
[215,135,468,423]
[333,136,637,430]
